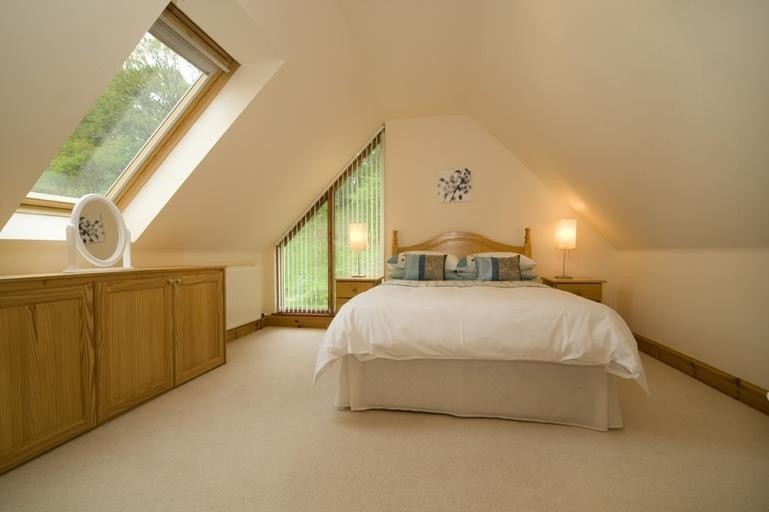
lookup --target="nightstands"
[334,276,385,315]
[540,275,606,303]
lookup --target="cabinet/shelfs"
[95,266,227,427]
[0,273,94,475]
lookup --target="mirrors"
[62,193,131,271]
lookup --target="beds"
[312,227,650,430]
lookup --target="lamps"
[554,218,578,281]
[349,224,368,278]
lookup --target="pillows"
[456,251,536,273]
[459,272,537,283]
[472,255,522,281]
[389,269,462,281]
[401,253,447,281]
[387,251,459,271]
